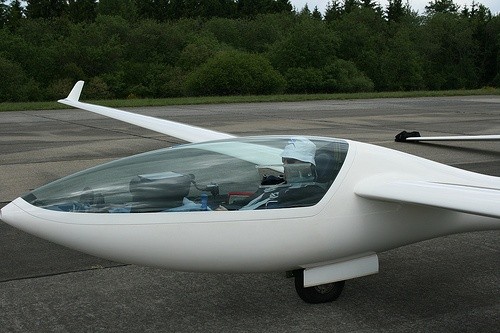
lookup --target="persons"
[216,135,327,211]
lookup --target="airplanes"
[1,78,496,306]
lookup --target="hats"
[281,136,317,166]
